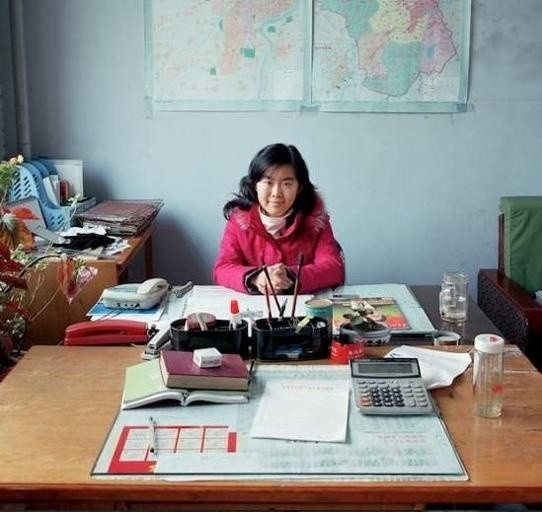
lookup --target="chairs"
[477,193,541,364]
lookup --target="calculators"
[349,358,433,415]
[142,327,171,360]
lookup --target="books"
[120,349,251,410]
[332,298,410,330]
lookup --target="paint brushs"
[263,253,304,326]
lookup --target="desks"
[1,343,541,512]
[89,284,508,345]
[10,222,157,345]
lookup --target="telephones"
[65,320,147,346]
[102,278,169,310]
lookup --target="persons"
[214,143,344,294]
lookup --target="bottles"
[228,300,242,329]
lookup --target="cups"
[438,271,470,323]
[471,334,505,419]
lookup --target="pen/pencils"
[184,313,208,331]
[149,417,155,452]
